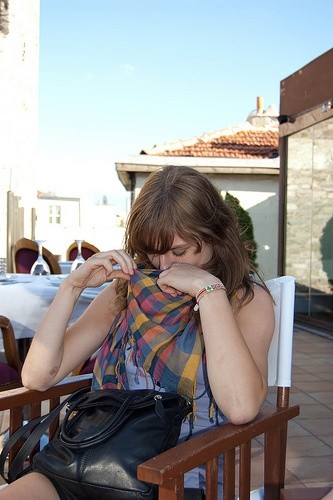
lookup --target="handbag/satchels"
[0,387,193,500]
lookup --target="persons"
[1,165,276,500]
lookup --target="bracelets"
[194,283,226,311]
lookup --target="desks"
[0,261,120,353]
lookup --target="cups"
[0,258,6,281]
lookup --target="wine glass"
[70,240,86,273]
[30,240,51,285]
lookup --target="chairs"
[1,316,23,391]
[0,275,299,500]
[11,237,62,274]
[66,241,101,261]
